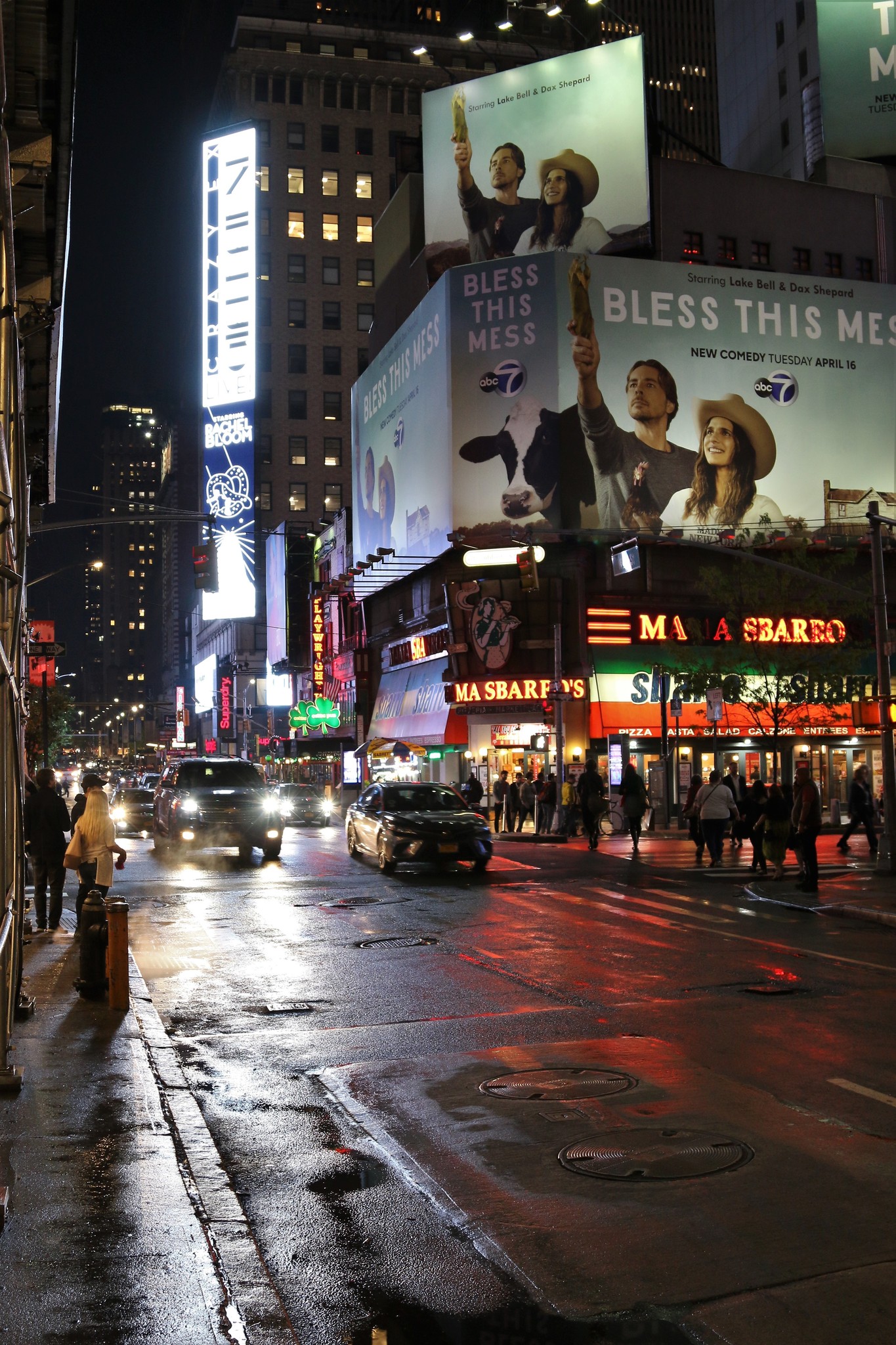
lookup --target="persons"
[55,779,62,798]
[681,774,705,864]
[565,316,699,531]
[787,768,822,891]
[744,780,768,875]
[72,790,127,941]
[750,765,760,784]
[619,392,793,546]
[464,772,484,807]
[493,149,618,259]
[753,784,790,881]
[451,126,540,263]
[619,763,652,852]
[836,764,878,855]
[693,769,740,867]
[70,774,109,839]
[373,455,398,557]
[22,768,72,934]
[354,440,380,562]
[24,781,38,798]
[492,768,577,837]
[601,765,609,793]
[722,762,747,847]
[576,758,606,851]
[61,776,72,798]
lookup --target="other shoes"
[837,838,851,849]
[36,926,48,932]
[749,866,756,870]
[759,869,768,875]
[795,880,818,892]
[47,923,68,934]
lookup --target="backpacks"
[538,781,551,801]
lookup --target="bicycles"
[575,796,624,837]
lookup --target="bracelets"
[755,823,759,826]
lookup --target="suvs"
[152,753,283,862]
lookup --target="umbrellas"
[353,736,426,759]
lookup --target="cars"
[51,751,161,832]
[345,781,494,875]
[270,784,331,826]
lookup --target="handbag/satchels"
[587,792,603,813]
[625,788,649,809]
[732,821,749,839]
[63,826,81,870]
[764,829,775,843]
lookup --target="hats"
[378,455,395,525]
[536,149,599,207]
[693,393,777,480]
[82,773,109,789]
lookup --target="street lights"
[213,678,256,760]
[89,705,138,763]
[113,698,136,766]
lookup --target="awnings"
[367,655,469,747]
[589,647,896,739]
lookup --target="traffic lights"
[177,710,183,722]
[193,542,218,592]
[530,735,549,752]
[516,546,540,593]
[243,720,249,730]
[543,700,556,726]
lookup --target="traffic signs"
[239,746,251,751]
[549,680,562,692]
[549,691,574,702]
[25,641,66,657]
[706,688,722,721]
